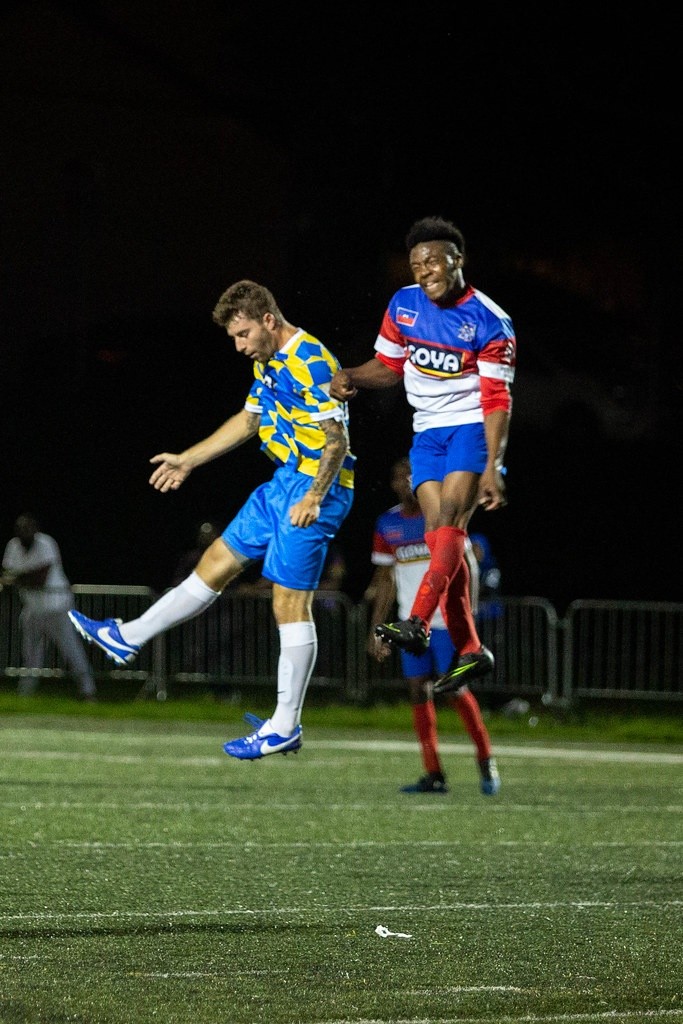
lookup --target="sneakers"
[432,643,495,695]
[373,616,432,658]
[220,712,303,761]
[67,609,141,672]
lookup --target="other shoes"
[399,772,451,793]
[477,756,501,796]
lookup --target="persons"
[366,461,500,795]
[329,218,517,693]
[469,537,505,692]
[0,513,96,699]
[66,280,358,760]
